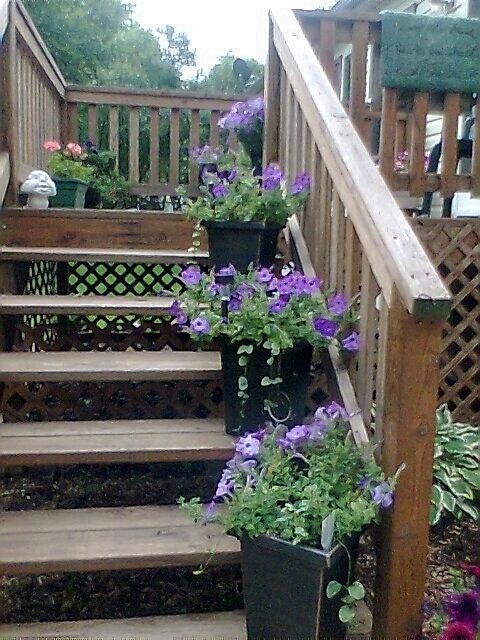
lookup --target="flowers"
[42,134,95,181]
[174,141,313,253]
[176,402,406,576]
[168,257,364,432]
[168,97,401,631]
[215,100,263,133]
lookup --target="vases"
[53,176,90,208]
[229,521,348,637]
[199,219,283,285]
[219,336,313,434]
[207,157,261,196]
